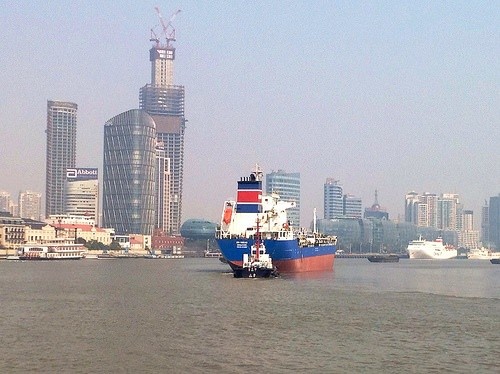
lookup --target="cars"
[335,249,344,256]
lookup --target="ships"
[404,235,458,261]
[212,160,339,273]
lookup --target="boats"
[18,241,87,262]
[227,211,281,279]
[367,252,400,264]
[490,258,500,264]
